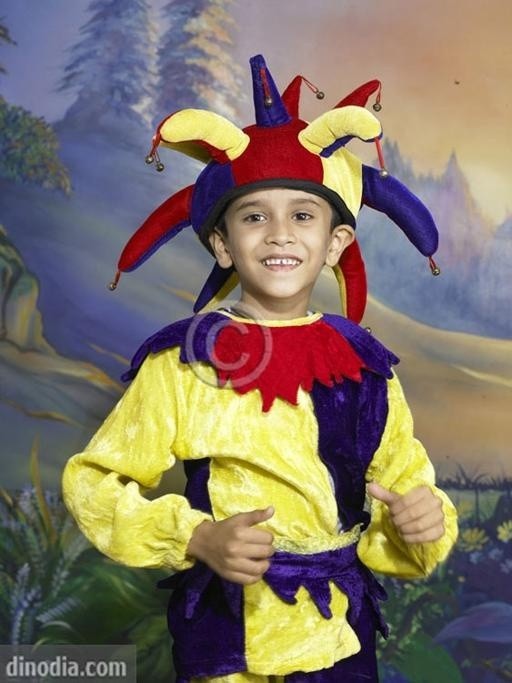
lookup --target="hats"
[108,56,440,323]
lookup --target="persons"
[61,187,460,683]
